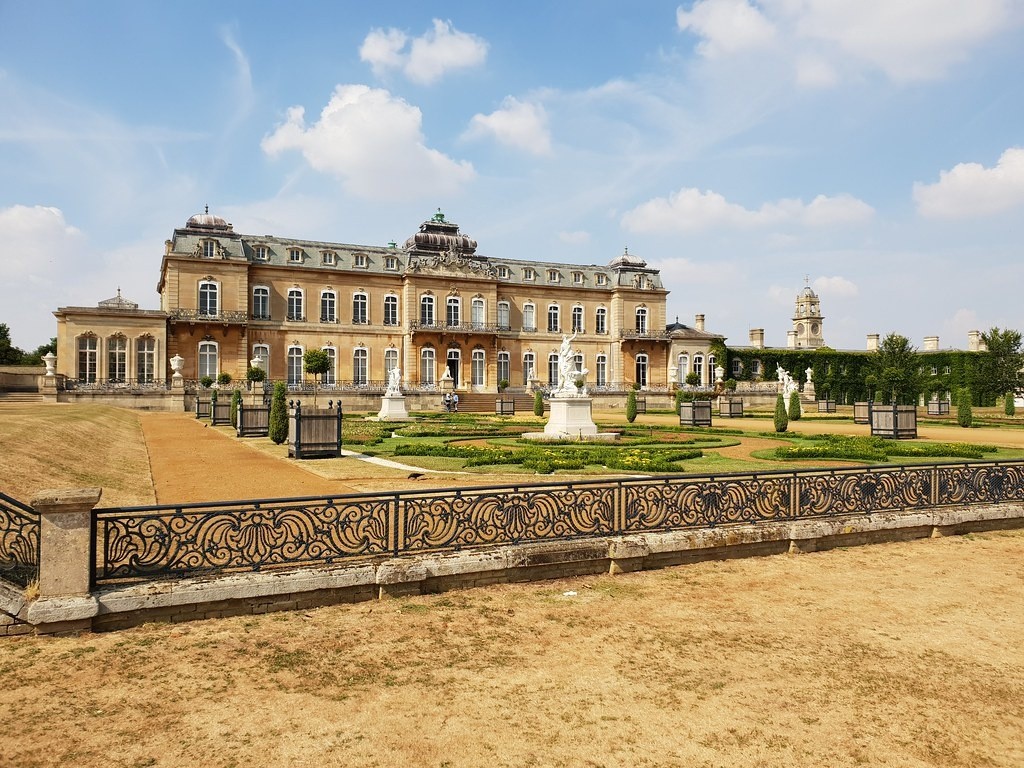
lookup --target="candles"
[626,382,647,415]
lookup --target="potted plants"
[195,375,213,420]
[718,377,743,419]
[871,366,919,441]
[495,378,515,416]
[210,370,232,427]
[928,376,952,417]
[817,382,837,414]
[236,366,271,439]
[854,374,882,423]
[678,372,713,426]
[287,347,343,459]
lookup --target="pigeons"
[408,473,425,479]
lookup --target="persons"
[549,368,590,397]
[559,327,578,372]
[446,391,459,412]
[388,366,401,393]
